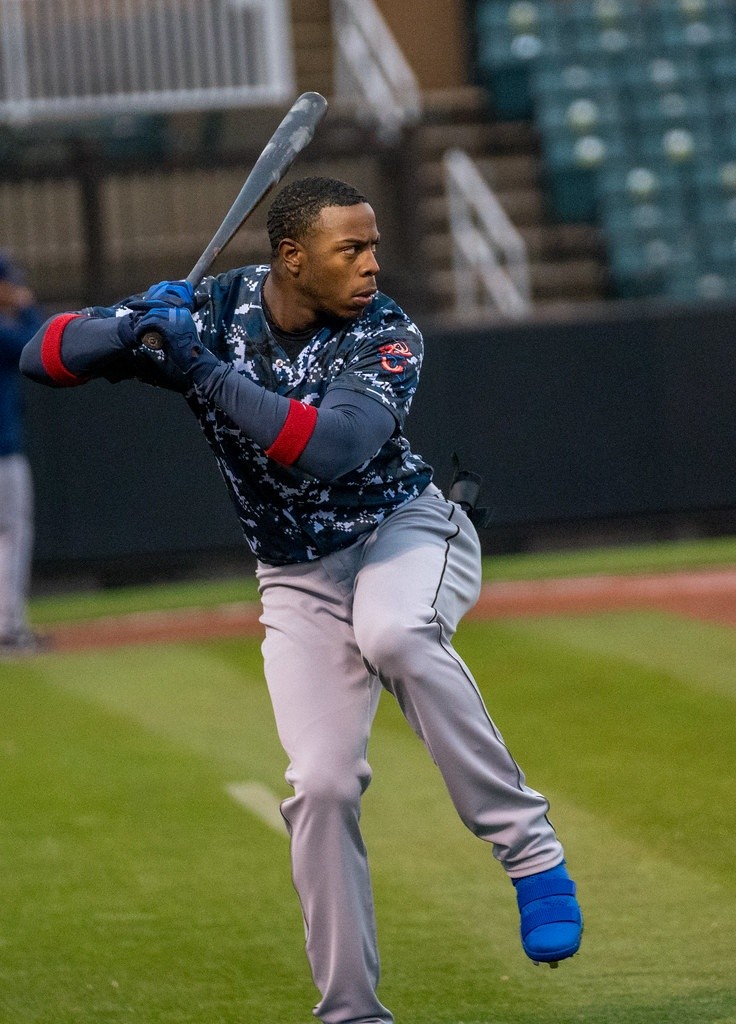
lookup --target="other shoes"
[0,631,41,654]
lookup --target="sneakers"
[511,858,584,969]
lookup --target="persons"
[0,256,48,643]
[16,176,583,1024]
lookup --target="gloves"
[126,299,203,365]
[131,280,209,329]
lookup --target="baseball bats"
[141,90,330,351]
